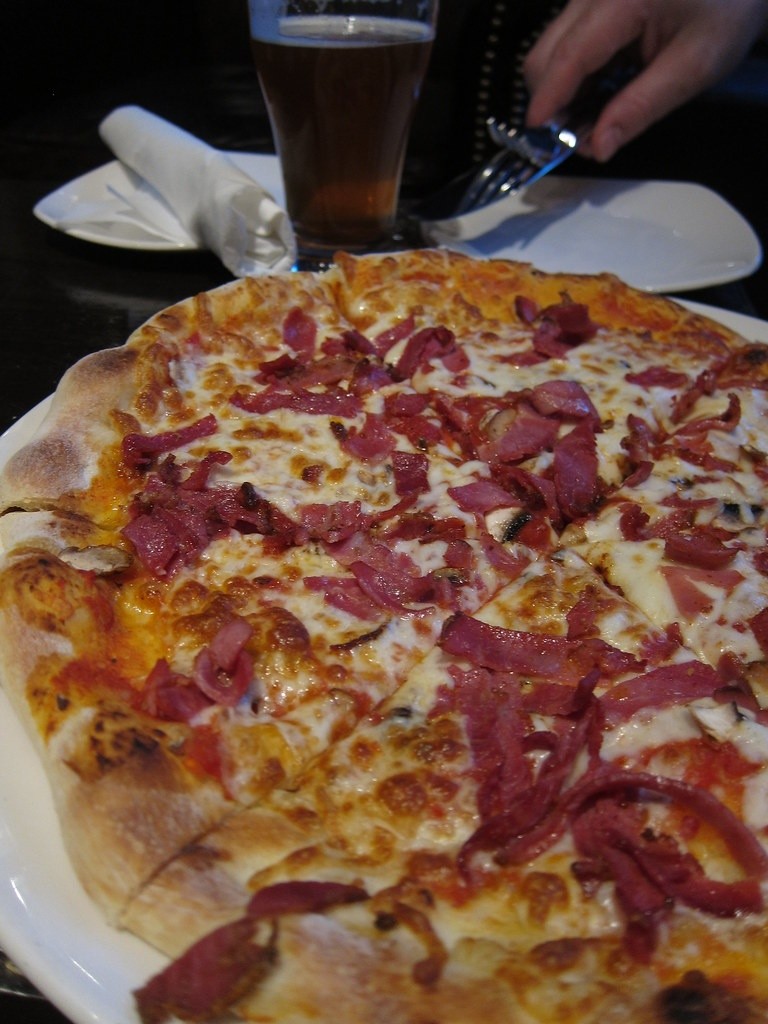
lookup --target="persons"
[522,0,767,159]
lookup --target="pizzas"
[0,246,768,1024]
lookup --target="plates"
[0,289,767,1024]
[35,151,289,252]
[422,175,760,293]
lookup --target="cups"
[248,2,436,261]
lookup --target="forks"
[457,149,544,211]
[485,107,596,168]
